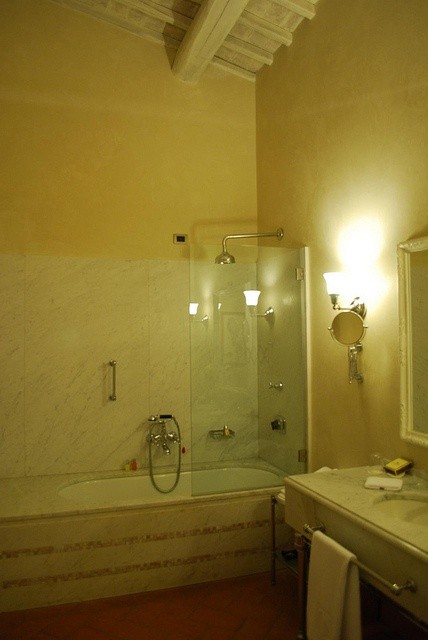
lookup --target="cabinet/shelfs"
[270,494,297,596]
[285,483,427,640]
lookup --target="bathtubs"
[57,466,283,503]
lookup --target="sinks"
[374,492,428,526]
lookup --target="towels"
[276,467,337,503]
[306,529,363,640]
[364,475,404,491]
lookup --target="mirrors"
[328,311,368,346]
[395,236,427,447]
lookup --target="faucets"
[147,431,177,456]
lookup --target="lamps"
[243,289,275,325]
[323,272,367,318]
[189,303,208,324]
